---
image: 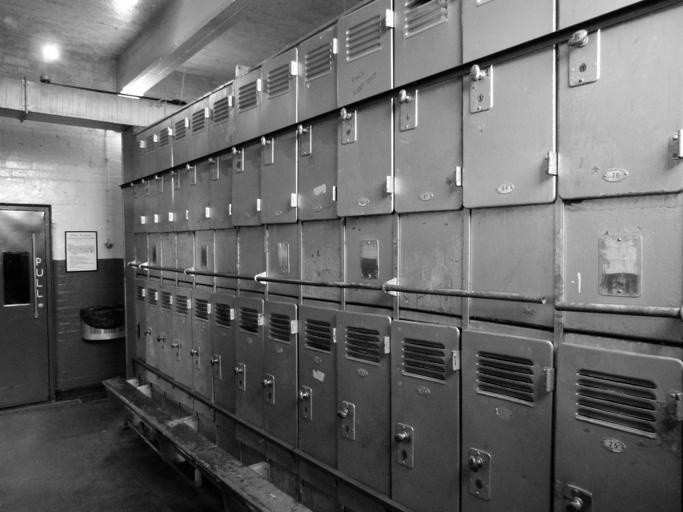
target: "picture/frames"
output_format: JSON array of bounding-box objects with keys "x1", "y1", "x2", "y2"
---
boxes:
[{"x1": 65, "y1": 229, "x2": 99, "y2": 273}]
